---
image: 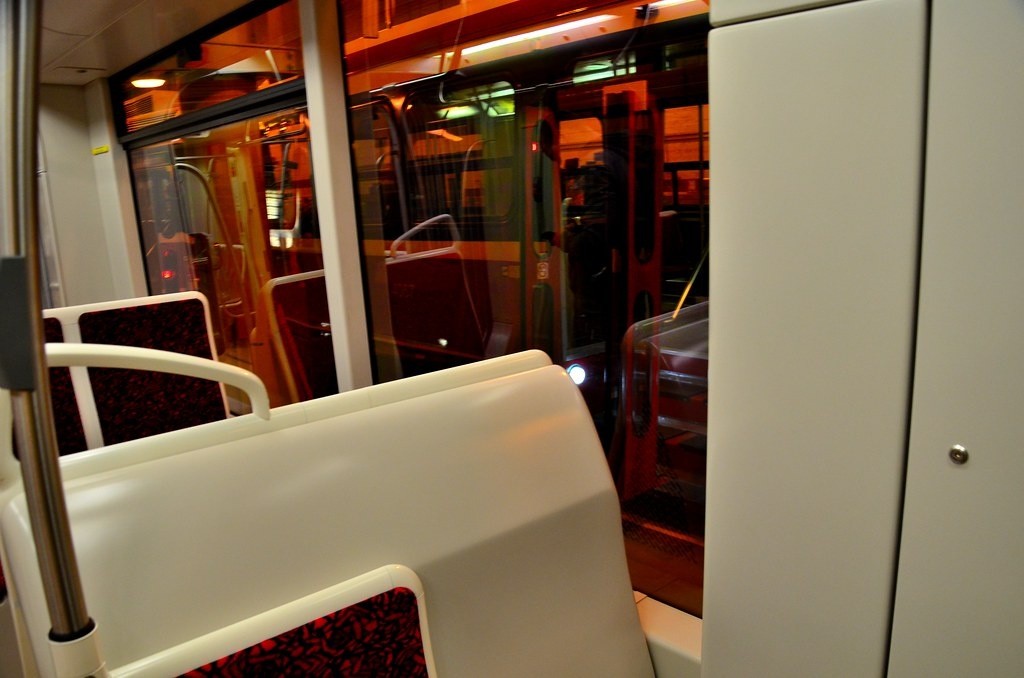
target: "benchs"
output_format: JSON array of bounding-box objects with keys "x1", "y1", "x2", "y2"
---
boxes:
[
  {"x1": 256, "y1": 214, "x2": 488, "y2": 412},
  {"x1": 11, "y1": 290, "x2": 231, "y2": 462},
  {"x1": 0, "y1": 346, "x2": 660, "y2": 678}
]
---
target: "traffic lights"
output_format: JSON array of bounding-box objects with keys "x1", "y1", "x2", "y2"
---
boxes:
[{"x1": 160, "y1": 233, "x2": 225, "y2": 355}]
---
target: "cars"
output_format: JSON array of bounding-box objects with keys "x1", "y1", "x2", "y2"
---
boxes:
[{"x1": 616, "y1": 248, "x2": 712, "y2": 451}]
